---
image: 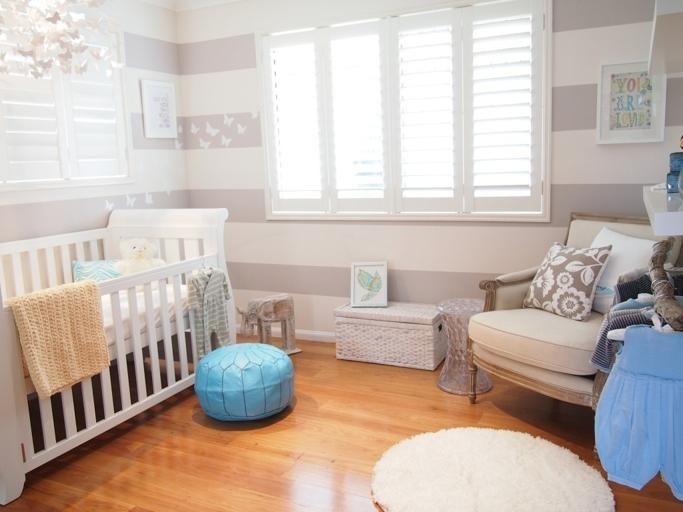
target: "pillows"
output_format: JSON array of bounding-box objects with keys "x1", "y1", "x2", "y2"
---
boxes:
[
  {"x1": 73, "y1": 259, "x2": 120, "y2": 281},
  {"x1": 522, "y1": 243, "x2": 612, "y2": 321},
  {"x1": 588, "y1": 225, "x2": 660, "y2": 315}
]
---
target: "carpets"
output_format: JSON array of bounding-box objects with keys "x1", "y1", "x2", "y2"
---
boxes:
[{"x1": 369, "y1": 428, "x2": 617, "y2": 512}]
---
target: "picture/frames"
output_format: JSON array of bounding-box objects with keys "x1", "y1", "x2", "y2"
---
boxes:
[
  {"x1": 595, "y1": 63, "x2": 667, "y2": 145},
  {"x1": 350, "y1": 262, "x2": 389, "y2": 307},
  {"x1": 142, "y1": 79, "x2": 178, "y2": 139}
]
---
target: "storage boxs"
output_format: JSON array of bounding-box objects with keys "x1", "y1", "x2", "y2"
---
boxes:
[{"x1": 334, "y1": 301, "x2": 447, "y2": 372}]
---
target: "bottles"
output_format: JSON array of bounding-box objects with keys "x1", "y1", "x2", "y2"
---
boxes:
[{"x1": 667, "y1": 152, "x2": 683, "y2": 210}]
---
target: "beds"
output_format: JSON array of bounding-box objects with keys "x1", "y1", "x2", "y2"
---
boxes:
[{"x1": 0, "y1": 208, "x2": 237, "y2": 505}]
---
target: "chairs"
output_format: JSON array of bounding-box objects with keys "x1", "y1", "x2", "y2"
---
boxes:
[{"x1": 467, "y1": 210, "x2": 683, "y2": 410}]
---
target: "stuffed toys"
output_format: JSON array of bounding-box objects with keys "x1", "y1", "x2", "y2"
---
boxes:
[{"x1": 113, "y1": 238, "x2": 158, "y2": 293}]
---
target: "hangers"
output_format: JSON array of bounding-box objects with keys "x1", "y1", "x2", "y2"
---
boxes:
[{"x1": 646, "y1": 238, "x2": 675, "y2": 326}]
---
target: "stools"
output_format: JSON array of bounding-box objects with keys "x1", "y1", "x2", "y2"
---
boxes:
[{"x1": 437, "y1": 299, "x2": 493, "y2": 394}]
